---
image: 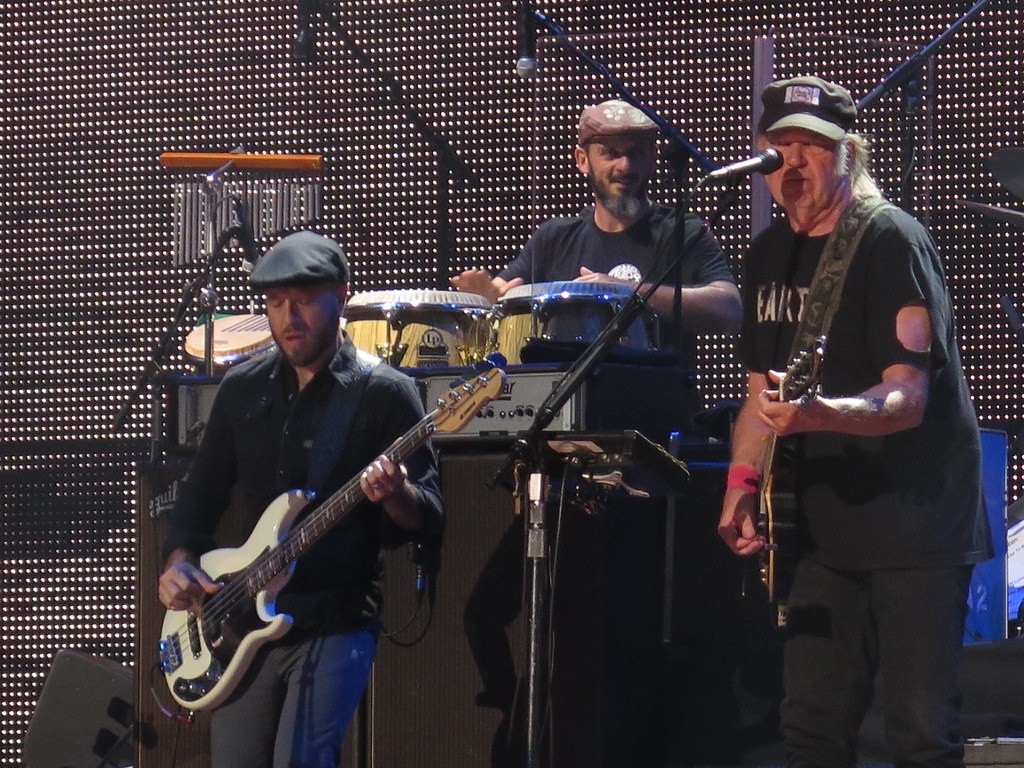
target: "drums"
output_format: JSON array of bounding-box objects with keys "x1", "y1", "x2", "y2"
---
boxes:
[
  {"x1": 491, "y1": 280, "x2": 659, "y2": 363},
  {"x1": 339, "y1": 288, "x2": 492, "y2": 370},
  {"x1": 182, "y1": 311, "x2": 275, "y2": 378}
]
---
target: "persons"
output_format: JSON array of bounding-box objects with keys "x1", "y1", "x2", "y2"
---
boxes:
[
  {"x1": 157, "y1": 231, "x2": 447, "y2": 768},
  {"x1": 448, "y1": 98, "x2": 744, "y2": 370},
  {"x1": 718, "y1": 75, "x2": 996, "y2": 767}
]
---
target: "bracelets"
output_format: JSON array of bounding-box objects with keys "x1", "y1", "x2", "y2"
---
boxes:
[{"x1": 727, "y1": 463, "x2": 762, "y2": 497}]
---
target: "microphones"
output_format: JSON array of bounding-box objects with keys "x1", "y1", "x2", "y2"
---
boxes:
[
  {"x1": 517, "y1": 0, "x2": 538, "y2": 79},
  {"x1": 707, "y1": 149, "x2": 784, "y2": 183},
  {"x1": 231, "y1": 196, "x2": 262, "y2": 272},
  {"x1": 294, "y1": 0, "x2": 317, "y2": 63}
]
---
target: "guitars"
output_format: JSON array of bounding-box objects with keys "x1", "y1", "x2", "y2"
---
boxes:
[
  {"x1": 733, "y1": 333, "x2": 829, "y2": 622},
  {"x1": 154, "y1": 353, "x2": 508, "y2": 713}
]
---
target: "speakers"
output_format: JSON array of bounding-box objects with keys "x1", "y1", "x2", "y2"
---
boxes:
[
  {"x1": 22, "y1": 649, "x2": 138, "y2": 768},
  {"x1": 136, "y1": 449, "x2": 606, "y2": 768}
]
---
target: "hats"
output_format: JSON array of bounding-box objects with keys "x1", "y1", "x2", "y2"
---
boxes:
[
  {"x1": 577, "y1": 98, "x2": 660, "y2": 145},
  {"x1": 247, "y1": 229, "x2": 351, "y2": 291},
  {"x1": 759, "y1": 76, "x2": 858, "y2": 141}
]
